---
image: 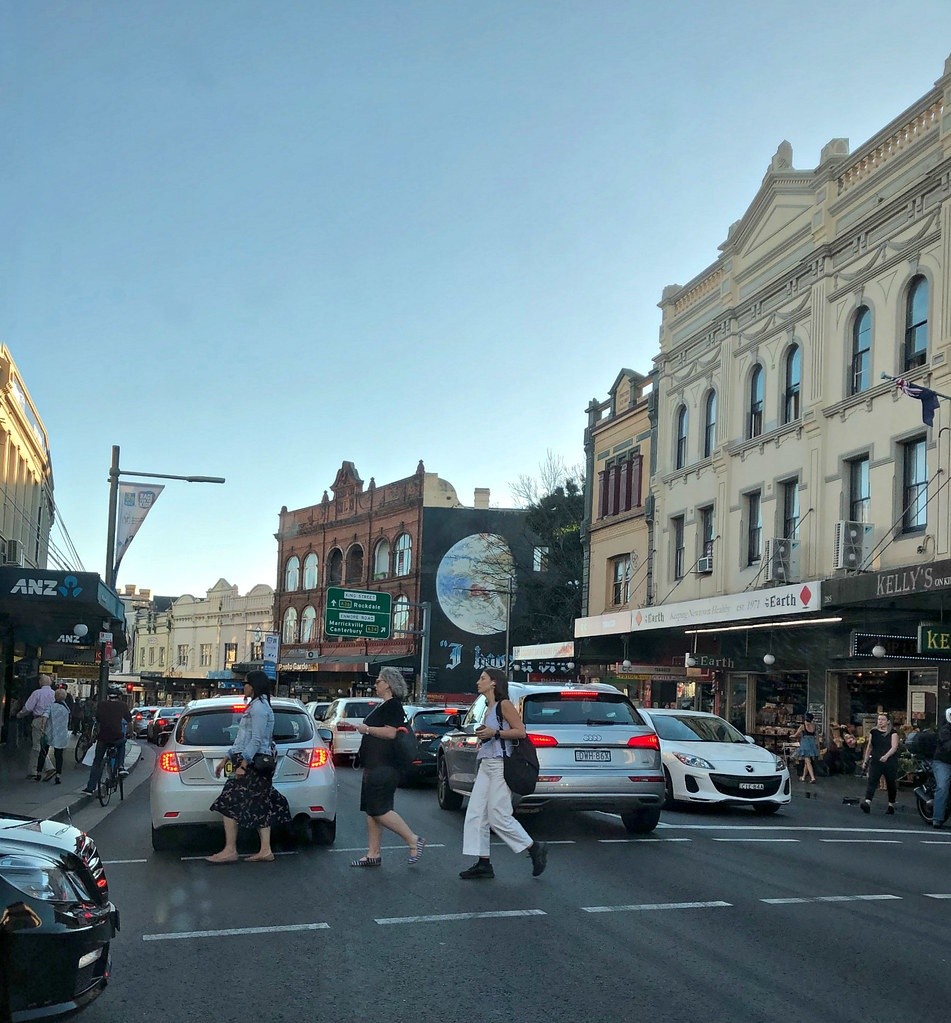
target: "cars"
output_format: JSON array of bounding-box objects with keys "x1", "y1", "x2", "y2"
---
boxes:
[
  {"x1": 0, "y1": 811, "x2": 121, "y2": 1023},
  {"x1": 315, "y1": 698, "x2": 385, "y2": 754},
  {"x1": 291, "y1": 701, "x2": 331, "y2": 735},
  {"x1": 402, "y1": 702, "x2": 472, "y2": 787},
  {"x1": 146, "y1": 707, "x2": 184, "y2": 746},
  {"x1": 130, "y1": 706, "x2": 160, "y2": 735},
  {"x1": 606, "y1": 708, "x2": 791, "y2": 813},
  {"x1": 149, "y1": 697, "x2": 338, "y2": 850}
]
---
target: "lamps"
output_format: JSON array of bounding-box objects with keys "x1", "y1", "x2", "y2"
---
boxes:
[
  {"x1": 687, "y1": 626, "x2": 696, "y2": 667},
  {"x1": 73, "y1": 616, "x2": 89, "y2": 637},
  {"x1": 623, "y1": 636, "x2": 631, "y2": 669},
  {"x1": 872, "y1": 604, "x2": 886, "y2": 658},
  {"x1": 763, "y1": 618, "x2": 775, "y2": 665}
]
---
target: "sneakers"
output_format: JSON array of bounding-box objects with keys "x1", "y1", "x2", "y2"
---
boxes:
[
  {"x1": 119, "y1": 767, "x2": 130, "y2": 775},
  {"x1": 459, "y1": 862, "x2": 495, "y2": 878},
  {"x1": 82, "y1": 788, "x2": 94, "y2": 795},
  {"x1": 526, "y1": 842, "x2": 548, "y2": 876}
]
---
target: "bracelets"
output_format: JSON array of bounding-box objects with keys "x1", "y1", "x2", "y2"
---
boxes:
[
  {"x1": 495, "y1": 730, "x2": 500, "y2": 740},
  {"x1": 240, "y1": 766, "x2": 247, "y2": 771},
  {"x1": 366, "y1": 728, "x2": 370, "y2": 735}
]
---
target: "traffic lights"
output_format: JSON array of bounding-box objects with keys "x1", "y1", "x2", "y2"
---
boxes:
[{"x1": 127, "y1": 684, "x2": 132, "y2": 694}]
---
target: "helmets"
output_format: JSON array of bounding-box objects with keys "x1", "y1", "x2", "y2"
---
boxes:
[{"x1": 945, "y1": 707, "x2": 951, "y2": 724}]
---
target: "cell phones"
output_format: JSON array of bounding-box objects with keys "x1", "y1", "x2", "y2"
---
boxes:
[{"x1": 475, "y1": 725, "x2": 491, "y2": 743}]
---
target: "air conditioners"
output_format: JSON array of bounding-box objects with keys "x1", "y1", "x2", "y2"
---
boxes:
[
  {"x1": 764, "y1": 538, "x2": 800, "y2": 585},
  {"x1": 697, "y1": 557, "x2": 713, "y2": 572},
  {"x1": 834, "y1": 520, "x2": 875, "y2": 572},
  {"x1": 305, "y1": 651, "x2": 318, "y2": 660},
  {"x1": 5, "y1": 540, "x2": 24, "y2": 568}
]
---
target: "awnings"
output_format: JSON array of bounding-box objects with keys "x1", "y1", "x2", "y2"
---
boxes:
[{"x1": 232, "y1": 654, "x2": 415, "y2": 673}]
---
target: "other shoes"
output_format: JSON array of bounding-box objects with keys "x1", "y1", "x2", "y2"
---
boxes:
[
  {"x1": 860, "y1": 802, "x2": 871, "y2": 813},
  {"x1": 798, "y1": 776, "x2": 805, "y2": 782},
  {"x1": 932, "y1": 818, "x2": 941, "y2": 829},
  {"x1": 809, "y1": 779, "x2": 816, "y2": 784},
  {"x1": 55, "y1": 776, "x2": 61, "y2": 784},
  {"x1": 408, "y1": 835, "x2": 426, "y2": 864},
  {"x1": 350, "y1": 855, "x2": 382, "y2": 865},
  {"x1": 886, "y1": 806, "x2": 896, "y2": 816},
  {"x1": 42, "y1": 770, "x2": 56, "y2": 781},
  {"x1": 243, "y1": 852, "x2": 274, "y2": 862},
  {"x1": 25, "y1": 774, "x2": 42, "y2": 782},
  {"x1": 205, "y1": 849, "x2": 239, "y2": 862}
]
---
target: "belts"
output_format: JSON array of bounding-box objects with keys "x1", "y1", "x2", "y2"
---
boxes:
[{"x1": 34, "y1": 715, "x2": 42, "y2": 718}]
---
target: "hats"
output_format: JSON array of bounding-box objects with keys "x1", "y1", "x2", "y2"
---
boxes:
[{"x1": 109, "y1": 689, "x2": 123, "y2": 698}]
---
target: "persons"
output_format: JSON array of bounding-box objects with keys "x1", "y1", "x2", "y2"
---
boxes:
[
  {"x1": 203, "y1": 670, "x2": 292, "y2": 863},
  {"x1": 81, "y1": 688, "x2": 132, "y2": 796},
  {"x1": 931, "y1": 707, "x2": 951, "y2": 828},
  {"x1": 458, "y1": 669, "x2": 547, "y2": 878},
  {"x1": 861, "y1": 713, "x2": 899, "y2": 815},
  {"x1": 17, "y1": 675, "x2": 83, "y2": 782},
  {"x1": 350, "y1": 666, "x2": 426, "y2": 866},
  {"x1": 790, "y1": 713, "x2": 820, "y2": 783}
]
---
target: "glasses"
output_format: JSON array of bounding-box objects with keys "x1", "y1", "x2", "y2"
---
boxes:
[
  {"x1": 375, "y1": 678, "x2": 387, "y2": 686},
  {"x1": 879, "y1": 711, "x2": 888, "y2": 716},
  {"x1": 241, "y1": 680, "x2": 250, "y2": 686}
]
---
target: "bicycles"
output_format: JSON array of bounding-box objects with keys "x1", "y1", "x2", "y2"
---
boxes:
[
  {"x1": 74, "y1": 715, "x2": 96, "y2": 762},
  {"x1": 97, "y1": 722, "x2": 123, "y2": 806}
]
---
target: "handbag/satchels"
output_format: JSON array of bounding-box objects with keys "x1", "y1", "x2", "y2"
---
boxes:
[
  {"x1": 389, "y1": 724, "x2": 420, "y2": 769},
  {"x1": 497, "y1": 697, "x2": 539, "y2": 796}
]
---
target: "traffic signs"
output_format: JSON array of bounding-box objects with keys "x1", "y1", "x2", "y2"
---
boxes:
[{"x1": 325, "y1": 587, "x2": 392, "y2": 640}]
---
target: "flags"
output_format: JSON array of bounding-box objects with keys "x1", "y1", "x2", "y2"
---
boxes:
[{"x1": 892, "y1": 376, "x2": 940, "y2": 426}]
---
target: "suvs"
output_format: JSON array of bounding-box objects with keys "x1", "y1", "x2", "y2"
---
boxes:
[{"x1": 437, "y1": 681, "x2": 665, "y2": 832}]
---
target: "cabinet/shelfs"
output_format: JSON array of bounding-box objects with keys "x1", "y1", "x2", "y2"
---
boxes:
[
  {"x1": 756, "y1": 674, "x2": 808, "y2": 705},
  {"x1": 845, "y1": 670, "x2": 887, "y2": 692}
]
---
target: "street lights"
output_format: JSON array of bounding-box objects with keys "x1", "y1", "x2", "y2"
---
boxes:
[
  {"x1": 98, "y1": 446, "x2": 225, "y2": 703},
  {"x1": 246, "y1": 630, "x2": 282, "y2": 698}
]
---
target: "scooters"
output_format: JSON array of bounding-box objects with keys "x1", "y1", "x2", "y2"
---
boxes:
[{"x1": 904, "y1": 733, "x2": 951, "y2": 824}]
